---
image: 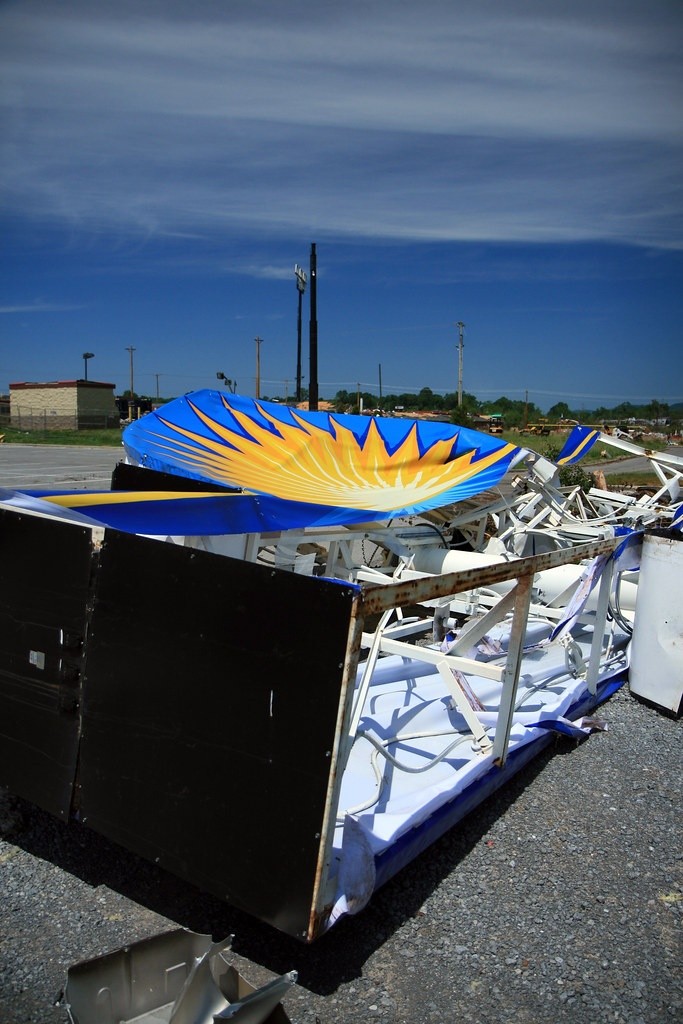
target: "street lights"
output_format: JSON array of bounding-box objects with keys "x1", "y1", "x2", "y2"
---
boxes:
[{"x1": 82, "y1": 352, "x2": 94, "y2": 382}]
